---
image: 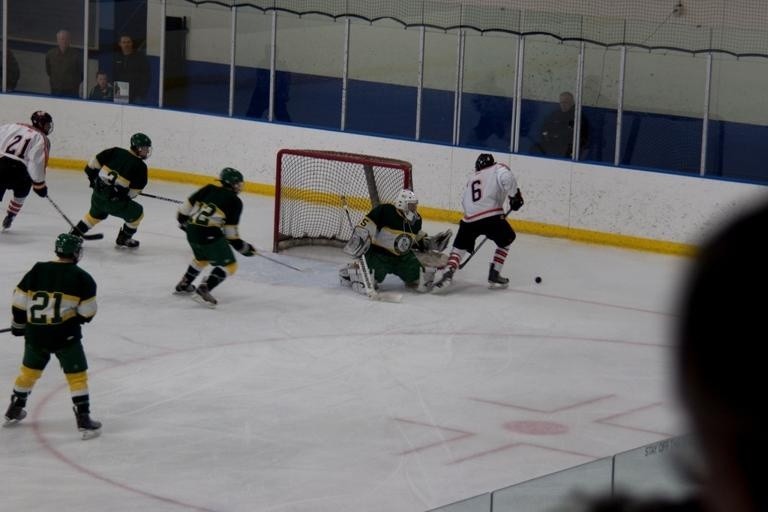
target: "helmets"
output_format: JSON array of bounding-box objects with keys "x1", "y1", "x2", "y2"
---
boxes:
[
  {"x1": 395, "y1": 189, "x2": 417, "y2": 221},
  {"x1": 131, "y1": 132, "x2": 151, "y2": 160},
  {"x1": 220, "y1": 167, "x2": 243, "y2": 189},
  {"x1": 55, "y1": 233, "x2": 83, "y2": 264},
  {"x1": 31, "y1": 110, "x2": 53, "y2": 134},
  {"x1": 475, "y1": 153, "x2": 494, "y2": 171}
]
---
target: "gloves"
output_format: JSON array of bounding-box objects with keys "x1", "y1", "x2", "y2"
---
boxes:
[
  {"x1": 239, "y1": 241, "x2": 254, "y2": 256},
  {"x1": 11, "y1": 321, "x2": 26, "y2": 336},
  {"x1": 33, "y1": 180, "x2": 47, "y2": 197},
  {"x1": 509, "y1": 188, "x2": 524, "y2": 211}
]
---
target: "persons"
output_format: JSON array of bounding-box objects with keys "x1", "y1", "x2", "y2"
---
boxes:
[
  {"x1": 174, "y1": 167, "x2": 255, "y2": 306},
  {"x1": 0, "y1": 51, "x2": 21, "y2": 91},
  {"x1": 112, "y1": 35, "x2": 151, "y2": 102},
  {"x1": 345, "y1": 186, "x2": 424, "y2": 285},
  {"x1": 438, "y1": 155, "x2": 526, "y2": 286},
  {"x1": 583, "y1": 192, "x2": 768, "y2": 512},
  {"x1": 532, "y1": 91, "x2": 586, "y2": 157},
  {"x1": 4, "y1": 233, "x2": 104, "y2": 433},
  {"x1": 45, "y1": 29, "x2": 86, "y2": 97},
  {"x1": 86, "y1": 73, "x2": 115, "y2": 101},
  {"x1": 0, "y1": 110, "x2": 53, "y2": 228},
  {"x1": 69, "y1": 131, "x2": 151, "y2": 248}
]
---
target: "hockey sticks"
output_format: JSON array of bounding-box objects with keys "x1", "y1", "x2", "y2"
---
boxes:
[
  {"x1": 46, "y1": 195, "x2": 103, "y2": 240},
  {"x1": 252, "y1": 251, "x2": 318, "y2": 275},
  {"x1": 436, "y1": 208, "x2": 512, "y2": 270},
  {"x1": 341, "y1": 196, "x2": 404, "y2": 302}
]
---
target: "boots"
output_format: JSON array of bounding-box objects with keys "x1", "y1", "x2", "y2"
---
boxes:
[
  {"x1": 487, "y1": 263, "x2": 508, "y2": 283},
  {"x1": 176, "y1": 276, "x2": 194, "y2": 292},
  {"x1": 5, "y1": 395, "x2": 26, "y2": 420},
  {"x1": 2, "y1": 213, "x2": 14, "y2": 228},
  {"x1": 69, "y1": 228, "x2": 80, "y2": 237},
  {"x1": 116, "y1": 227, "x2": 138, "y2": 247},
  {"x1": 436, "y1": 266, "x2": 455, "y2": 288},
  {"x1": 72, "y1": 406, "x2": 101, "y2": 429},
  {"x1": 196, "y1": 277, "x2": 216, "y2": 304}
]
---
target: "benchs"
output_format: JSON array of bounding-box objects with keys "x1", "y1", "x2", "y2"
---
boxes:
[{"x1": 98, "y1": 53, "x2": 722, "y2": 182}]
